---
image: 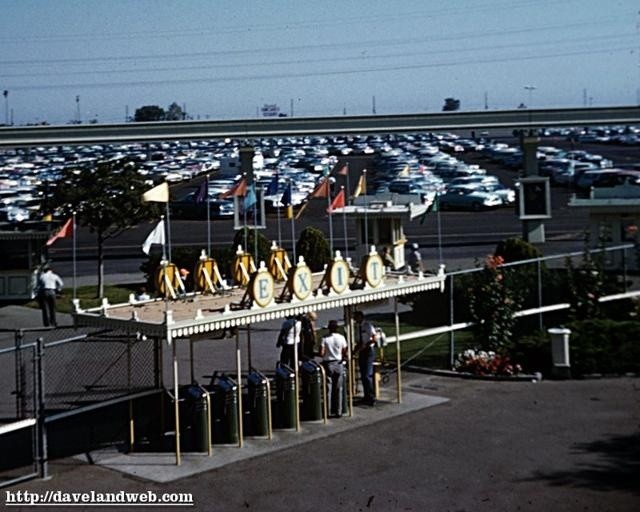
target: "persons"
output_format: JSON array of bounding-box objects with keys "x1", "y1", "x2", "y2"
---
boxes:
[
  {"x1": 351, "y1": 310, "x2": 376, "y2": 407},
  {"x1": 276, "y1": 312, "x2": 316, "y2": 369},
  {"x1": 26, "y1": 259, "x2": 64, "y2": 328},
  {"x1": 318, "y1": 320, "x2": 348, "y2": 418}
]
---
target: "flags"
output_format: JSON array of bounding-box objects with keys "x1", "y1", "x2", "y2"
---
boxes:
[
  {"x1": 44, "y1": 216, "x2": 73, "y2": 247},
  {"x1": 215, "y1": 175, "x2": 293, "y2": 219},
  {"x1": 141, "y1": 222, "x2": 165, "y2": 257},
  {"x1": 140, "y1": 181, "x2": 170, "y2": 205},
  {"x1": 311, "y1": 164, "x2": 365, "y2": 215},
  {"x1": 397, "y1": 163, "x2": 425, "y2": 177},
  {"x1": 191, "y1": 177, "x2": 208, "y2": 204}
]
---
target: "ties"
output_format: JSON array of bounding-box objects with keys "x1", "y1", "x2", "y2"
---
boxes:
[{"x1": 359, "y1": 324, "x2": 362, "y2": 342}]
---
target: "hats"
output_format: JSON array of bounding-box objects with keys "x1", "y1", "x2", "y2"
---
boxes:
[{"x1": 328, "y1": 320, "x2": 340, "y2": 329}]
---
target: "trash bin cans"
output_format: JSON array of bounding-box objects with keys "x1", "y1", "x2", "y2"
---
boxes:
[{"x1": 546, "y1": 327, "x2": 573, "y2": 367}]
